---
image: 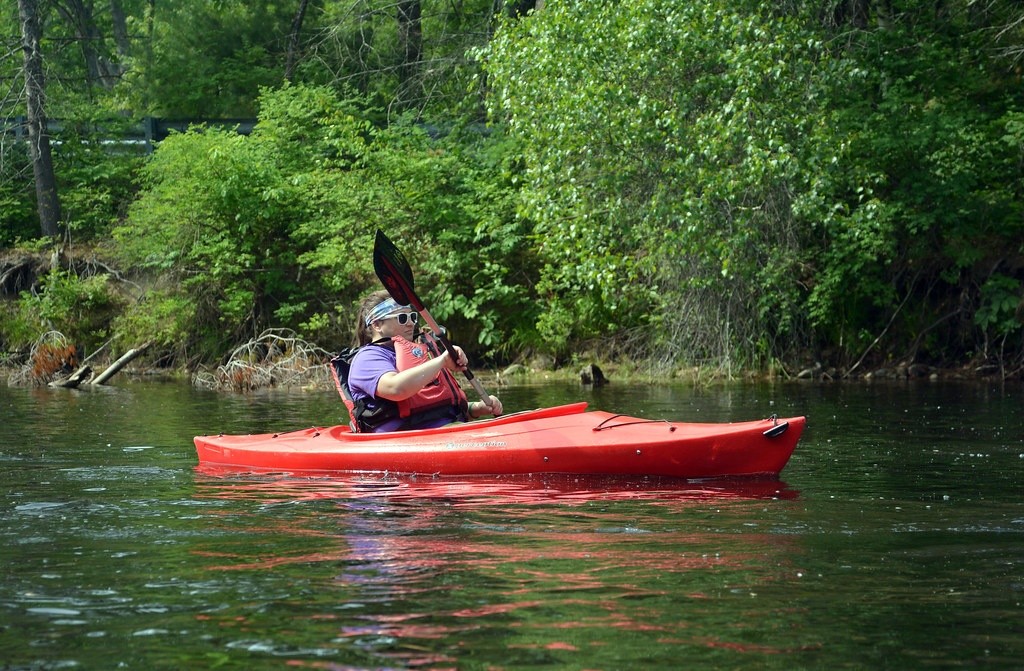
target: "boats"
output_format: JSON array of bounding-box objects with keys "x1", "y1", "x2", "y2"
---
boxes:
[{"x1": 193, "y1": 402, "x2": 806, "y2": 476}]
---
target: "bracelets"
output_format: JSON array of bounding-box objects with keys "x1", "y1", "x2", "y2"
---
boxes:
[{"x1": 468, "y1": 401, "x2": 480, "y2": 419}]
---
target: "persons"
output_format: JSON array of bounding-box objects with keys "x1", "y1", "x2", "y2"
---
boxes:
[{"x1": 345, "y1": 290, "x2": 501, "y2": 431}]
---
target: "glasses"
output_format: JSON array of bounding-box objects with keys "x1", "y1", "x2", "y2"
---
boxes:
[{"x1": 379, "y1": 312, "x2": 418, "y2": 326}]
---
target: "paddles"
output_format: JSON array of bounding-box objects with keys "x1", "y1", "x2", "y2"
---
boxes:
[{"x1": 372, "y1": 227, "x2": 498, "y2": 416}]
